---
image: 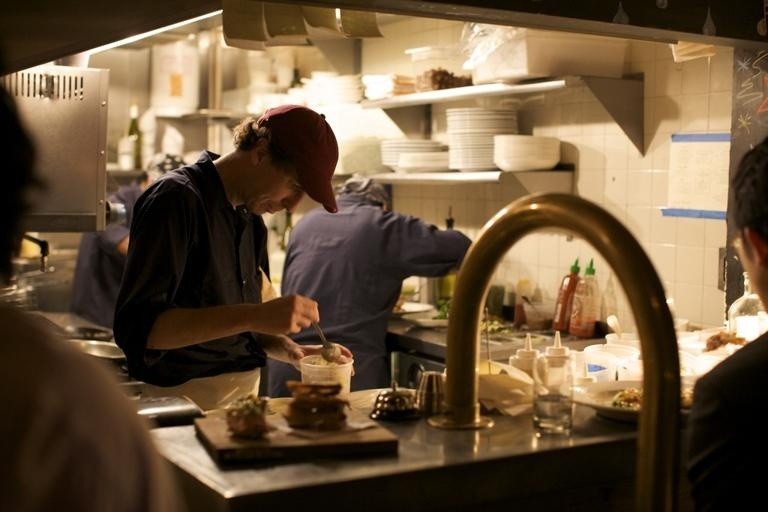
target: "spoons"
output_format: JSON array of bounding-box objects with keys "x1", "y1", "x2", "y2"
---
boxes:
[{"x1": 313, "y1": 322, "x2": 341, "y2": 362}]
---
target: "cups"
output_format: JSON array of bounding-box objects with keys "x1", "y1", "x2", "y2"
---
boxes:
[{"x1": 532, "y1": 356, "x2": 575, "y2": 438}]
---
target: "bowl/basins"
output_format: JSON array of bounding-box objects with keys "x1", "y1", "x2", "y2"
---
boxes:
[{"x1": 66, "y1": 327, "x2": 128, "y2": 368}]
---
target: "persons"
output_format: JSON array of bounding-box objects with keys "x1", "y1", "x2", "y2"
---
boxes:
[
  {"x1": 683, "y1": 136, "x2": 767, "y2": 510},
  {"x1": 111, "y1": 107, "x2": 358, "y2": 408},
  {"x1": 70, "y1": 152, "x2": 189, "y2": 326},
  {"x1": 263, "y1": 177, "x2": 474, "y2": 401},
  {"x1": 0, "y1": 82, "x2": 193, "y2": 512}
]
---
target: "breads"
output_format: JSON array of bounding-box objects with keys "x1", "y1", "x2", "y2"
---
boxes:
[
  {"x1": 225, "y1": 394, "x2": 268, "y2": 437},
  {"x1": 285, "y1": 395, "x2": 347, "y2": 429}
]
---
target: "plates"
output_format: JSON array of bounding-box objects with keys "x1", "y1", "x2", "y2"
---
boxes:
[
  {"x1": 571, "y1": 379, "x2": 695, "y2": 424},
  {"x1": 380, "y1": 106, "x2": 561, "y2": 173},
  {"x1": 400, "y1": 312, "x2": 448, "y2": 329}
]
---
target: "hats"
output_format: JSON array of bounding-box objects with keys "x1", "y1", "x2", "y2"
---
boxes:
[{"x1": 255, "y1": 104, "x2": 340, "y2": 214}]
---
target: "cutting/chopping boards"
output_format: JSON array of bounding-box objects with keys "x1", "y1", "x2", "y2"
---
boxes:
[{"x1": 194, "y1": 407, "x2": 399, "y2": 465}]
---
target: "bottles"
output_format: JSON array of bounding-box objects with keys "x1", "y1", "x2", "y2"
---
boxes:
[
  {"x1": 126, "y1": 104, "x2": 144, "y2": 168},
  {"x1": 551, "y1": 257, "x2": 599, "y2": 336},
  {"x1": 510, "y1": 330, "x2": 570, "y2": 385}
]
